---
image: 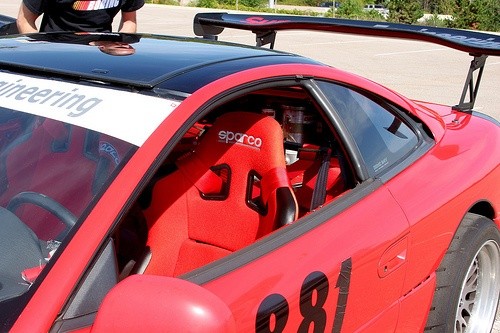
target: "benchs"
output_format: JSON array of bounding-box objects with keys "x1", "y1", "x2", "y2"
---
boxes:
[{"x1": 168, "y1": 93, "x2": 358, "y2": 219}]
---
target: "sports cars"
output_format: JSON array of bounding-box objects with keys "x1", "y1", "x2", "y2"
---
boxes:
[{"x1": 0, "y1": 9, "x2": 500, "y2": 333}]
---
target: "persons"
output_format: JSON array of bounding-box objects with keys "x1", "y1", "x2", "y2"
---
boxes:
[
  {"x1": 24, "y1": 31, "x2": 143, "y2": 56},
  {"x1": 16, "y1": 0, "x2": 145, "y2": 34}
]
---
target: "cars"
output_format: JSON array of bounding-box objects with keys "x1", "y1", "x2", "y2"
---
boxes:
[{"x1": 363, "y1": 4, "x2": 384, "y2": 15}]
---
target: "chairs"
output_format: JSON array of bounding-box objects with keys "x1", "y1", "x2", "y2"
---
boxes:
[
  {"x1": 142, "y1": 109, "x2": 298, "y2": 277},
  {"x1": 1, "y1": 118, "x2": 103, "y2": 245}
]
